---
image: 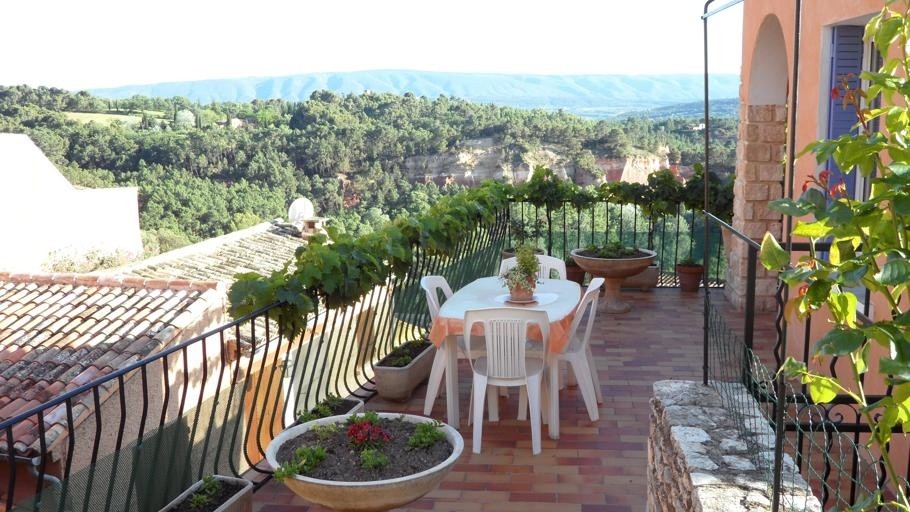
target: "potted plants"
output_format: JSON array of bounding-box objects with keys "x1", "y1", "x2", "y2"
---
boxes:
[
  {"x1": 265, "y1": 411, "x2": 465, "y2": 512},
  {"x1": 157, "y1": 470, "x2": 255, "y2": 512},
  {"x1": 501, "y1": 243, "x2": 542, "y2": 304},
  {"x1": 282, "y1": 395, "x2": 365, "y2": 434},
  {"x1": 572, "y1": 240, "x2": 657, "y2": 314},
  {"x1": 675, "y1": 255, "x2": 703, "y2": 293},
  {"x1": 564, "y1": 255, "x2": 586, "y2": 284},
  {"x1": 374, "y1": 338, "x2": 436, "y2": 403},
  {"x1": 501, "y1": 220, "x2": 546, "y2": 257}
]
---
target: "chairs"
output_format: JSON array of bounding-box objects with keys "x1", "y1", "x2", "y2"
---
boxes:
[
  {"x1": 497, "y1": 255, "x2": 569, "y2": 281},
  {"x1": 462, "y1": 307, "x2": 551, "y2": 457},
  {"x1": 518, "y1": 275, "x2": 607, "y2": 424},
  {"x1": 420, "y1": 274, "x2": 501, "y2": 429}
]
